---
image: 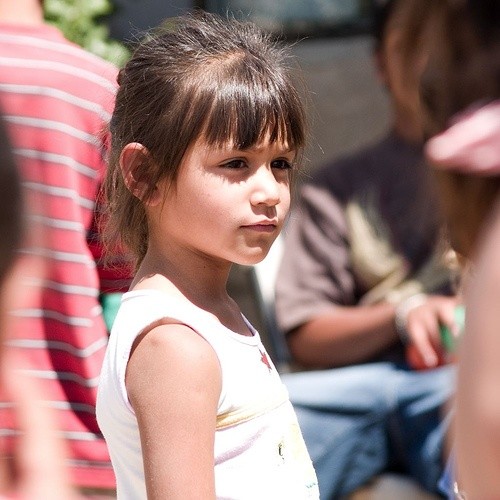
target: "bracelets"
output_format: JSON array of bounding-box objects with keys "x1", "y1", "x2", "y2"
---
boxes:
[{"x1": 395, "y1": 293, "x2": 425, "y2": 340}]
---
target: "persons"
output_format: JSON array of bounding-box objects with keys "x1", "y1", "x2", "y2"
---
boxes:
[
  {"x1": 91, "y1": 10, "x2": 325, "y2": 500},
  {"x1": 266, "y1": 0, "x2": 500, "y2": 500},
  {"x1": 1, "y1": 1, "x2": 129, "y2": 500}
]
